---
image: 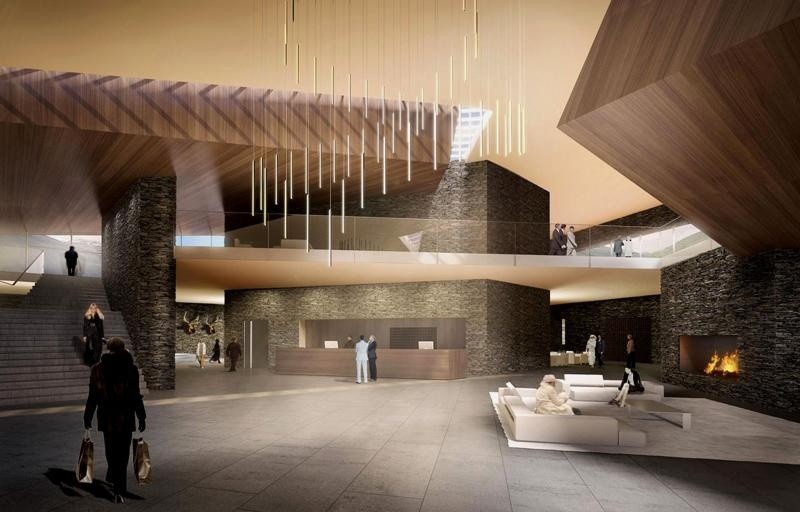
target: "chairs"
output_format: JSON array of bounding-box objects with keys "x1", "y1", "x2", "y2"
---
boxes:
[{"x1": 550, "y1": 351, "x2": 589, "y2": 367}]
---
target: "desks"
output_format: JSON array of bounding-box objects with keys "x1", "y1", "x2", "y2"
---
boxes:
[{"x1": 625, "y1": 400, "x2": 692, "y2": 432}]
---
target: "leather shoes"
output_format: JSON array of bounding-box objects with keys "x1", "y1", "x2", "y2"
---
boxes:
[{"x1": 114, "y1": 494, "x2": 127, "y2": 504}]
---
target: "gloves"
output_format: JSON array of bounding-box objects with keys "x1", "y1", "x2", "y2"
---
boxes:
[
  {"x1": 84, "y1": 419, "x2": 92, "y2": 430},
  {"x1": 138, "y1": 418, "x2": 146, "y2": 432}
]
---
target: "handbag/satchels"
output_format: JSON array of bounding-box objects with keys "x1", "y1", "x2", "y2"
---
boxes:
[
  {"x1": 73, "y1": 430, "x2": 95, "y2": 484},
  {"x1": 132, "y1": 430, "x2": 153, "y2": 488}
]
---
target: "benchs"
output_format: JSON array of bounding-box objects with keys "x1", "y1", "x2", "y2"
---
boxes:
[{"x1": 616, "y1": 417, "x2": 646, "y2": 447}]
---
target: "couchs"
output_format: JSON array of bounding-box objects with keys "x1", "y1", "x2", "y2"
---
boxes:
[
  {"x1": 497, "y1": 387, "x2": 618, "y2": 446},
  {"x1": 555, "y1": 373, "x2": 665, "y2": 401}
]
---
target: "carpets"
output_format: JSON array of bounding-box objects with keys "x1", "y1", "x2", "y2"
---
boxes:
[{"x1": 490, "y1": 391, "x2": 800, "y2": 465}]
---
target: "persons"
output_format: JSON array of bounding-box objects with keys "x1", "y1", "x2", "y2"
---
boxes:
[
  {"x1": 226, "y1": 336, "x2": 242, "y2": 372},
  {"x1": 65, "y1": 246, "x2": 78, "y2": 276},
  {"x1": 355, "y1": 335, "x2": 369, "y2": 384},
  {"x1": 624, "y1": 236, "x2": 633, "y2": 257},
  {"x1": 596, "y1": 334, "x2": 607, "y2": 368},
  {"x1": 196, "y1": 339, "x2": 207, "y2": 368},
  {"x1": 613, "y1": 235, "x2": 624, "y2": 257},
  {"x1": 83, "y1": 337, "x2": 146, "y2": 505},
  {"x1": 547, "y1": 223, "x2": 578, "y2": 256},
  {"x1": 367, "y1": 335, "x2": 378, "y2": 382},
  {"x1": 626, "y1": 334, "x2": 636, "y2": 368},
  {"x1": 84, "y1": 303, "x2": 107, "y2": 366},
  {"x1": 210, "y1": 339, "x2": 222, "y2": 364},
  {"x1": 534, "y1": 374, "x2": 576, "y2": 415},
  {"x1": 586, "y1": 334, "x2": 597, "y2": 368},
  {"x1": 344, "y1": 336, "x2": 354, "y2": 348},
  {"x1": 609, "y1": 366, "x2": 645, "y2": 408}
]
